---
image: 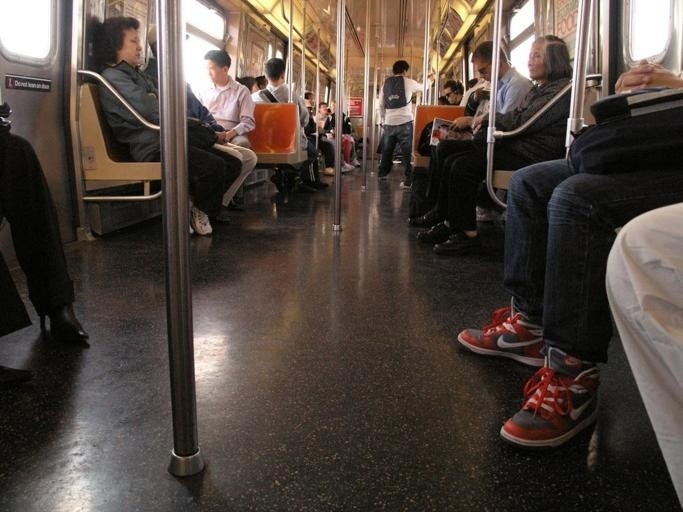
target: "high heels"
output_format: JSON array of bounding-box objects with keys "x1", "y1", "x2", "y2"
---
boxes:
[
  {"x1": 40, "y1": 305, "x2": 89, "y2": 342},
  {"x1": 1, "y1": 367, "x2": 36, "y2": 385}
]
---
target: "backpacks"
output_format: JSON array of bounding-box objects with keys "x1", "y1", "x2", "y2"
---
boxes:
[{"x1": 384, "y1": 76, "x2": 412, "y2": 110}]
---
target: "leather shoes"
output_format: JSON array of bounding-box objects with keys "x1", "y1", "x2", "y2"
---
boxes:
[{"x1": 408, "y1": 215, "x2": 482, "y2": 255}]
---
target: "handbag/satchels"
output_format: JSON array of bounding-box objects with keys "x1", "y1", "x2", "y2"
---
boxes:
[{"x1": 565, "y1": 86, "x2": 683, "y2": 176}]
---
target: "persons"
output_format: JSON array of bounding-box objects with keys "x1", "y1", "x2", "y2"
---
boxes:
[
  {"x1": 458, "y1": 24, "x2": 683, "y2": 451},
  {"x1": 376, "y1": 60, "x2": 435, "y2": 188},
  {"x1": 407, "y1": 34, "x2": 573, "y2": 255},
  {"x1": 1, "y1": 131, "x2": 90, "y2": 379},
  {"x1": 93, "y1": 16, "x2": 362, "y2": 234},
  {"x1": 606, "y1": 202, "x2": 683, "y2": 508}
]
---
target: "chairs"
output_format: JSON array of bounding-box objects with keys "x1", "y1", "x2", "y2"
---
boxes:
[
  {"x1": 352, "y1": 126, "x2": 370, "y2": 148},
  {"x1": 75, "y1": 81, "x2": 167, "y2": 201},
  {"x1": 248, "y1": 100, "x2": 309, "y2": 205},
  {"x1": 489, "y1": 86, "x2": 601, "y2": 192},
  {"x1": 410, "y1": 104, "x2": 465, "y2": 169}
]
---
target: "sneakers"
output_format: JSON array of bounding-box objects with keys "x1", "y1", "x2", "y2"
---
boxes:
[
  {"x1": 191, "y1": 206, "x2": 213, "y2": 236},
  {"x1": 299, "y1": 159, "x2": 360, "y2": 192},
  {"x1": 457, "y1": 297, "x2": 544, "y2": 368},
  {"x1": 499, "y1": 347, "x2": 600, "y2": 453}
]
